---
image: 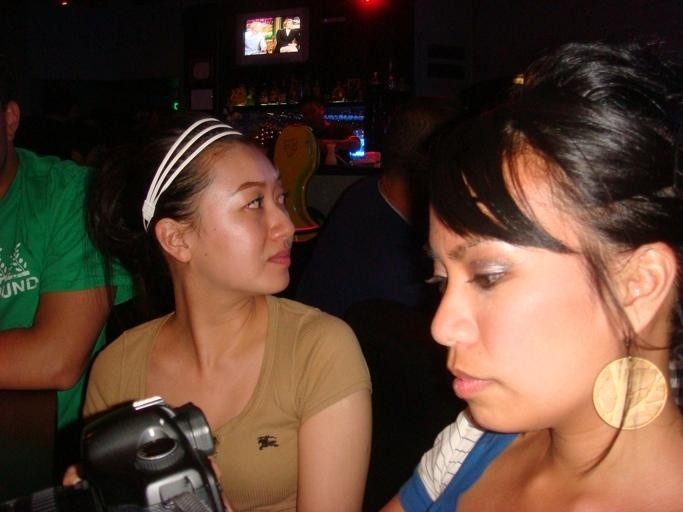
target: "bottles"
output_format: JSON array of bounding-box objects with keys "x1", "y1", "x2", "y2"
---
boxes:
[
  {"x1": 383, "y1": 63, "x2": 397, "y2": 90},
  {"x1": 254, "y1": 123, "x2": 275, "y2": 146},
  {"x1": 244, "y1": 77, "x2": 365, "y2": 105},
  {"x1": 324, "y1": 109, "x2": 366, "y2": 124},
  {"x1": 368, "y1": 71, "x2": 381, "y2": 86}
]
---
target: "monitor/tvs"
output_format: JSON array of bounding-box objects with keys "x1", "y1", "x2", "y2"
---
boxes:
[{"x1": 234, "y1": 6, "x2": 309, "y2": 67}]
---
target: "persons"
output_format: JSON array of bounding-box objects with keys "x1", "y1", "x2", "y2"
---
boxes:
[
  {"x1": 299, "y1": 94, "x2": 360, "y2": 166},
  {"x1": 60, "y1": 114, "x2": 374, "y2": 512},
  {"x1": 297, "y1": 72, "x2": 466, "y2": 512},
  {"x1": 0, "y1": 47, "x2": 135, "y2": 506},
  {"x1": 374, "y1": 29, "x2": 682, "y2": 512},
  {"x1": 94, "y1": 38, "x2": 188, "y2": 139}
]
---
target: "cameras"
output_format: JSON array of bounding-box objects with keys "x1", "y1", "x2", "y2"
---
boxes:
[{"x1": 0, "y1": 394, "x2": 227, "y2": 512}]
